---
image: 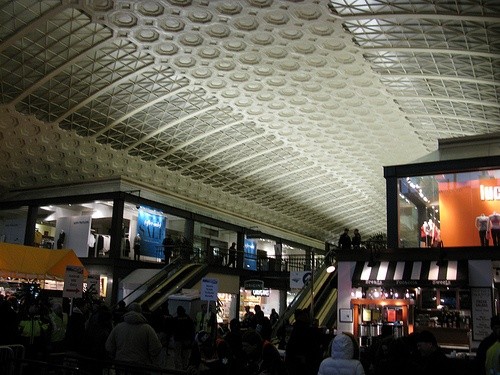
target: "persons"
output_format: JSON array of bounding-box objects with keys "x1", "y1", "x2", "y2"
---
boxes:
[
  {"x1": 289, "y1": 306, "x2": 329, "y2": 375},
  {"x1": 171, "y1": 306, "x2": 196, "y2": 373},
  {"x1": 196, "y1": 302, "x2": 278, "y2": 346},
  {"x1": 242, "y1": 330, "x2": 288, "y2": 375},
  {"x1": 18, "y1": 316, "x2": 49, "y2": 361},
  {"x1": 105, "y1": 303, "x2": 162, "y2": 375},
  {"x1": 124, "y1": 232, "x2": 130, "y2": 256},
  {"x1": 366, "y1": 316, "x2": 500, "y2": 375},
  {"x1": 339, "y1": 228, "x2": 351, "y2": 249},
  {"x1": 421, "y1": 219, "x2": 441, "y2": 247},
  {"x1": 133, "y1": 229, "x2": 145, "y2": 261},
  {"x1": 225, "y1": 242, "x2": 236, "y2": 268},
  {"x1": 475, "y1": 214, "x2": 490, "y2": 246},
  {"x1": 57, "y1": 229, "x2": 65, "y2": 249},
  {"x1": 159, "y1": 310, "x2": 173, "y2": 356},
  {"x1": 46, "y1": 303, "x2": 68, "y2": 356},
  {"x1": 318, "y1": 332, "x2": 365, "y2": 375},
  {"x1": 163, "y1": 233, "x2": 173, "y2": 264},
  {"x1": 352, "y1": 229, "x2": 361, "y2": 249},
  {"x1": 88, "y1": 230, "x2": 95, "y2": 257},
  {"x1": 44, "y1": 231, "x2": 48, "y2": 238},
  {"x1": 487, "y1": 211, "x2": 500, "y2": 246},
  {"x1": 64, "y1": 301, "x2": 89, "y2": 362},
  {"x1": 79, "y1": 314, "x2": 113, "y2": 375},
  {"x1": 0, "y1": 271, "x2": 127, "y2": 321}
]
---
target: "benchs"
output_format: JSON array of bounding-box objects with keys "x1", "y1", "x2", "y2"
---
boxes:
[{"x1": 415, "y1": 325, "x2": 473, "y2": 353}]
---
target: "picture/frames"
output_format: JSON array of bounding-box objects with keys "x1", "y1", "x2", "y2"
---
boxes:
[{"x1": 339, "y1": 308, "x2": 353, "y2": 323}]
